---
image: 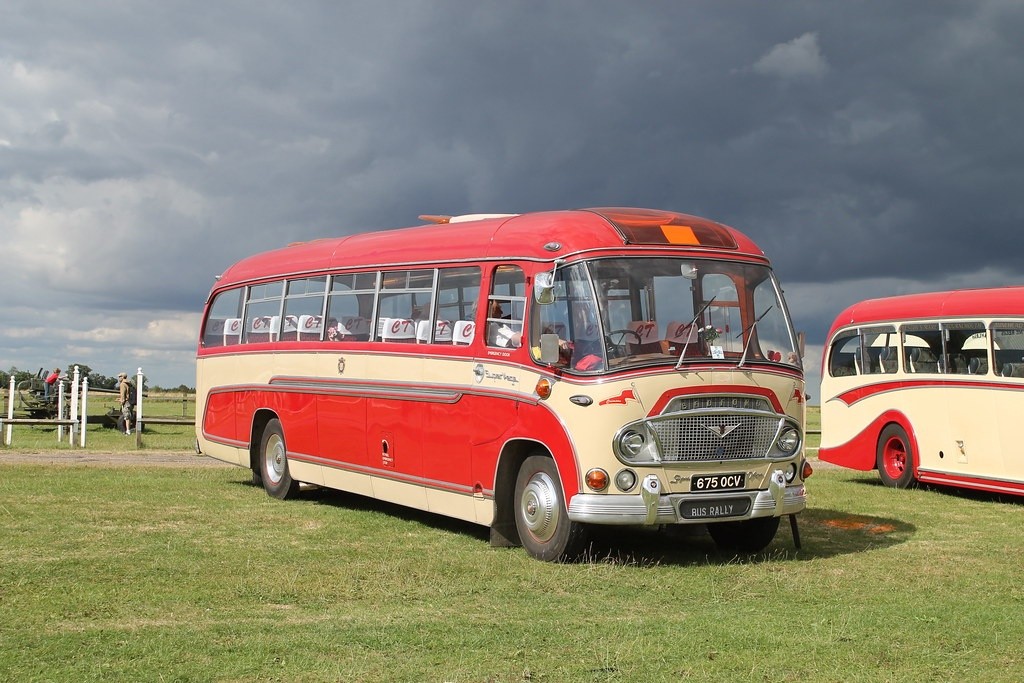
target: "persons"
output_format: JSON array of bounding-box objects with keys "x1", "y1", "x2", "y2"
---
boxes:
[
  {"x1": 42, "y1": 368, "x2": 61, "y2": 405},
  {"x1": 472, "y1": 298, "x2": 521, "y2": 348},
  {"x1": 116, "y1": 371, "x2": 138, "y2": 436}
]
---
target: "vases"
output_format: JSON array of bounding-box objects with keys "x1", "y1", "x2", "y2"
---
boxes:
[{"x1": 704, "y1": 339, "x2": 711, "y2": 356}]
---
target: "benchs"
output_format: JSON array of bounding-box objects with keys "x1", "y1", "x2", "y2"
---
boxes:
[
  {"x1": 1, "y1": 418, "x2": 80, "y2": 448},
  {"x1": 832, "y1": 356, "x2": 857, "y2": 377}
]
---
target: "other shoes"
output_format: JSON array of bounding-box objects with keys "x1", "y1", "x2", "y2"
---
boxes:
[{"x1": 123, "y1": 431, "x2": 131, "y2": 435}]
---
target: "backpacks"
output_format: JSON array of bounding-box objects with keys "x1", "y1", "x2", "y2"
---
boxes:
[{"x1": 124, "y1": 381, "x2": 137, "y2": 405}]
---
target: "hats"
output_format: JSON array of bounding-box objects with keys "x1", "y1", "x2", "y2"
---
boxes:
[{"x1": 117, "y1": 373, "x2": 127, "y2": 379}]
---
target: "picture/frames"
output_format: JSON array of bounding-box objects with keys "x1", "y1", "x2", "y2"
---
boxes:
[{"x1": 710, "y1": 346, "x2": 725, "y2": 359}]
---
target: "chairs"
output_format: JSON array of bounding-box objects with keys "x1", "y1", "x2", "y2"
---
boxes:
[
  {"x1": 327, "y1": 316, "x2": 344, "y2": 341},
  {"x1": 937, "y1": 353, "x2": 968, "y2": 374},
  {"x1": 574, "y1": 321, "x2": 603, "y2": 368},
  {"x1": 338, "y1": 316, "x2": 369, "y2": 342},
  {"x1": 378, "y1": 317, "x2": 391, "y2": 341},
  {"x1": 968, "y1": 357, "x2": 1001, "y2": 375},
  {"x1": 244, "y1": 315, "x2": 272, "y2": 343},
  {"x1": 878, "y1": 347, "x2": 910, "y2": 374},
  {"x1": 453, "y1": 320, "x2": 476, "y2": 346},
  {"x1": 1002, "y1": 362, "x2": 1024, "y2": 378},
  {"x1": 297, "y1": 315, "x2": 323, "y2": 342},
  {"x1": 854, "y1": 347, "x2": 881, "y2": 375},
  {"x1": 665, "y1": 321, "x2": 708, "y2": 355},
  {"x1": 416, "y1": 319, "x2": 452, "y2": 345},
  {"x1": 541, "y1": 320, "x2": 566, "y2": 340},
  {"x1": 204, "y1": 319, "x2": 229, "y2": 349},
  {"x1": 223, "y1": 318, "x2": 252, "y2": 346},
  {"x1": 624, "y1": 322, "x2": 664, "y2": 356},
  {"x1": 269, "y1": 315, "x2": 298, "y2": 342},
  {"x1": 909, "y1": 348, "x2": 939, "y2": 373},
  {"x1": 382, "y1": 318, "x2": 416, "y2": 344}
]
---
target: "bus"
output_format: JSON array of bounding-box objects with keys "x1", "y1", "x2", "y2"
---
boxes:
[
  {"x1": 817, "y1": 287, "x2": 1024, "y2": 498},
  {"x1": 193, "y1": 207, "x2": 815, "y2": 564}
]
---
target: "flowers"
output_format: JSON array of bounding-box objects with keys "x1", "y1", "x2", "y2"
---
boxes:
[{"x1": 699, "y1": 327, "x2": 723, "y2": 342}]
